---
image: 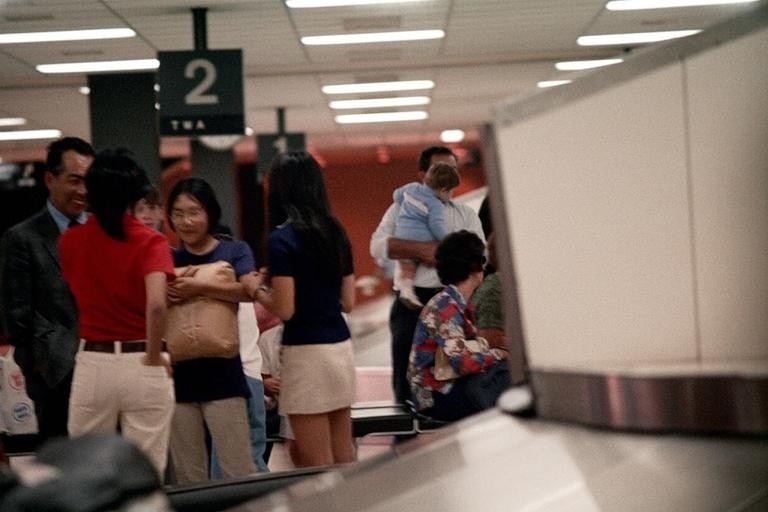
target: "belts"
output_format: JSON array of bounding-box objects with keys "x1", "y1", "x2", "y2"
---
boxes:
[{"x1": 83, "y1": 340, "x2": 168, "y2": 354}]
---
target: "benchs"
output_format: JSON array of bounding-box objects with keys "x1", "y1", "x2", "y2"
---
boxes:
[{"x1": 263, "y1": 399, "x2": 451, "y2": 467}]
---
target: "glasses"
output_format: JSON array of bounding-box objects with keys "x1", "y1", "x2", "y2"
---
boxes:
[{"x1": 170, "y1": 208, "x2": 206, "y2": 225}]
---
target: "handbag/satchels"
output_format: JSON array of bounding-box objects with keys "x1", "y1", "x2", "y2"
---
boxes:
[{"x1": 161, "y1": 260, "x2": 241, "y2": 365}]
